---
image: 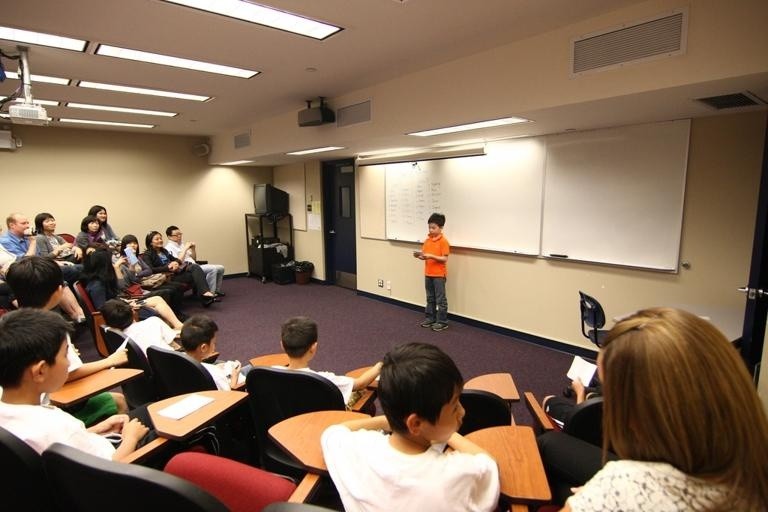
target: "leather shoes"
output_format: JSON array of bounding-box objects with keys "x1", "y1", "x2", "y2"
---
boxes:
[
  {"x1": 198, "y1": 291, "x2": 217, "y2": 298},
  {"x1": 199, "y1": 300, "x2": 212, "y2": 308}
]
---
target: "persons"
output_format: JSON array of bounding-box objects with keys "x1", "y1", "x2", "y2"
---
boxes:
[
  {"x1": 414, "y1": 213, "x2": 451, "y2": 332},
  {"x1": 542, "y1": 375, "x2": 603, "y2": 434},
  {"x1": 271, "y1": 314, "x2": 384, "y2": 413},
  {"x1": 1, "y1": 204, "x2": 233, "y2": 464},
  {"x1": 318, "y1": 339, "x2": 502, "y2": 512},
  {"x1": 558, "y1": 306, "x2": 768, "y2": 511}
]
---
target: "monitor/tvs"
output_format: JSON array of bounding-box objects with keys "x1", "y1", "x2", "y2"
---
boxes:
[{"x1": 252, "y1": 184, "x2": 288, "y2": 214}]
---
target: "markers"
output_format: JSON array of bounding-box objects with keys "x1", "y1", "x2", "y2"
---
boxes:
[{"x1": 549, "y1": 254, "x2": 568, "y2": 258}]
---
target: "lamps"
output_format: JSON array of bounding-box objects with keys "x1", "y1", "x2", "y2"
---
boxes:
[{"x1": 355, "y1": 139, "x2": 487, "y2": 167}]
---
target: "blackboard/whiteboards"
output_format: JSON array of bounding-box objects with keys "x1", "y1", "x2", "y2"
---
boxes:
[{"x1": 361, "y1": 118, "x2": 691, "y2": 275}]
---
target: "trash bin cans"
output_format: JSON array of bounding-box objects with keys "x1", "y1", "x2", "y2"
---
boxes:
[{"x1": 294, "y1": 270, "x2": 309, "y2": 284}]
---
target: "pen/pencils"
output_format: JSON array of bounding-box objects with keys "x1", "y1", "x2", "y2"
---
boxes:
[{"x1": 226, "y1": 364, "x2": 240, "y2": 379}]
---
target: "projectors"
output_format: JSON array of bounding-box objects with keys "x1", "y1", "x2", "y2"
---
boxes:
[{"x1": 9, "y1": 45, "x2": 52, "y2": 128}]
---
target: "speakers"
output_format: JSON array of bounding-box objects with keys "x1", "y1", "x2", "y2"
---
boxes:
[{"x1": 297, "y1": 107, "x2": 335, "y2": 126}]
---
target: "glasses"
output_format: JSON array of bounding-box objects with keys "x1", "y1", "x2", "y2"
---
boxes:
[{"x1": 171, "y1": 234, "x2": 182, "y2": 236}]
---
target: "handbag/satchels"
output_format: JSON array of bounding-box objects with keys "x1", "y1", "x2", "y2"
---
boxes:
[
  {"x1": 141, "y1": 274, "x2": 165, "y2": 289},
  {"x1": 125, "y1": 285, "x2": 143, "y2": 295},
  {"x1": 56, "y1": 248, "x2": 82, "y2": 264}
]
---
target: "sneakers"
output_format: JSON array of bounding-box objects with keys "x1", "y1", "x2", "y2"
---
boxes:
[
  {"x1": 433, "y1": 322, "x2": 449, "y2": 331},
  {"x1": 214, "y1": 298, "x2": 222, "y2": 302},
  {"x1": 215, "y1": 290, "x2": 225, "y2": 296},
  {"x1": 420, "y1": 319, "x2": 436, "y2": 327}
]
